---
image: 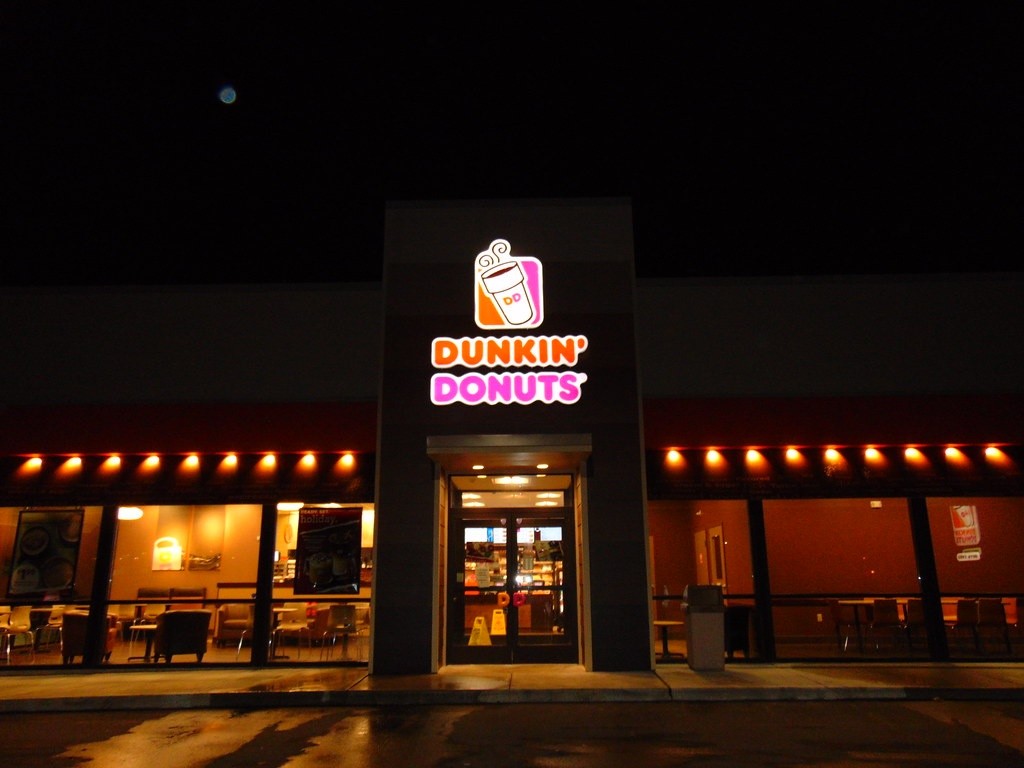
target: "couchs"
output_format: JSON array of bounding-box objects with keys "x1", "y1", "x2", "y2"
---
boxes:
[
  {"x1": 62, "y1": 610, "x2": 117, "y2": 664},
  {"x1": 152, "y1": 609, "x2": 212, "y2": 663},
  {"x1": 215, "y1": 604, "x2": 255, "y2": 649}
]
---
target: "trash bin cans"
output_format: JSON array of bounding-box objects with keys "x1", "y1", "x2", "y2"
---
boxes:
[{"x1": 681, "y1": 582, "x2": 727, "y2": 670}]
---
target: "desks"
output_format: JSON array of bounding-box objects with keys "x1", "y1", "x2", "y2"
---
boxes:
[
  {"x1": 654, "y1": 620, "x2": 685, "y2": 661},
  {"x1": 270, "y1": 608, "x2": 298, "y2": 660},
  {"x1": 128, "y1": 625, "x2": 165, "y2": 662},
  {"x1": 840, "y1": 600, "x2": 1010, "y2": 646}
]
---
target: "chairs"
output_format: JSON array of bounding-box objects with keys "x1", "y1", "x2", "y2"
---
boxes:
[
  {"x1": 724, "y1": 604, "x2": 752, "y2": 659},
  {"x1": 830, "y1": 598, "x2": 1011, "y2": 652},
  {"x1": 236, "y1": 601, "x2": 368, "y2": 661},
  {"x1": 0, "y1": 606, "x2": 89, "y2": 664},
  {"x1": 110, "y1": 615, "x2": 135, "y2": 642}
]
